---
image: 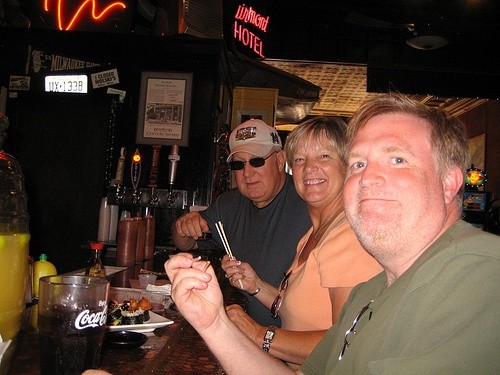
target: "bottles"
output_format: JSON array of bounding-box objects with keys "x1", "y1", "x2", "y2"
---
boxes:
[
  {"x1": 30, "y1": 254, "x2": 57, "y2": 331},
  {"x1": 116, "y1": 211, "x2": 155, "y2": 266},
  {"x1": 84, "y1": 242, "x2": 106, "y2": 279},
  {"x1": 0, "y1": 113, "x2": 31, "y2": 375}
]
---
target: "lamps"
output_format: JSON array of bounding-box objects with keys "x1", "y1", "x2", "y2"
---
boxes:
[{"x1": 406, "y1": 0, "x2": 459, "y2": 50}]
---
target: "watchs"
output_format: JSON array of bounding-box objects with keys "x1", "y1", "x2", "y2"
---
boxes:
[{"x1": 262, "y1": 325, "x2": 277, "y2": 354}]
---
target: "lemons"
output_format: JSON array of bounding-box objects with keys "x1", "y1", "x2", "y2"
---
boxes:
[{"x1": 32, "y1": 254, "x2": 57, "y2": 296}]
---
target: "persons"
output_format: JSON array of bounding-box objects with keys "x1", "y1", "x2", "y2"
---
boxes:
[
  {"x1": 173, "y1": 117, "x2": 311, "y2": 327},
  {"x1": 164, "y1": 92, "x2": 500, "y2": 375},
  {"x1": 221, "y1": 114, "x2": 384, "y2": 369}
]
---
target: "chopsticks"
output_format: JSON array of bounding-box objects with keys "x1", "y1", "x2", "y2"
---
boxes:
[
  {"x1": 215, "y1": 221, "x2": 244, "y2": 290},
  {"x1": 193, "y1": 255, "x2": 210, "y2": 272}
]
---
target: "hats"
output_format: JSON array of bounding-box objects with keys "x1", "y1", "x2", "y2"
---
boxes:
[{"x1": 225, "y1": 118, "x2": 282, "y2": 163}]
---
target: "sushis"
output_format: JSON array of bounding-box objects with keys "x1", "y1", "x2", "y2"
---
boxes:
[{"x1": 107, "y1": 297, "x2": 153, "y2": 325}]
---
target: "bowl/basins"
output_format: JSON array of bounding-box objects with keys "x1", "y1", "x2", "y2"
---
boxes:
[{"x1": 104, "y1": 331, "x2": 148, "y2": 351}]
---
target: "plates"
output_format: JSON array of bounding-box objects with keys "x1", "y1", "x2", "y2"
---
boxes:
[{"x1": 106, "y1": 303, "x2": 175, "y2": 333}]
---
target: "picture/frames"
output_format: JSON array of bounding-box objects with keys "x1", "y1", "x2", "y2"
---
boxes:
[{"x1": 136, "y1": 70, "x2": 194, "y2": 146}]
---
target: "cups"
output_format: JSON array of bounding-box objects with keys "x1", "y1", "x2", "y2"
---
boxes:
[
  {"x1": 138, "y1": 274, "x2": 157, "y2": 288},
  {"x1": 98, "y1": 197, "x2": 129, "y2": 243},
  {"x1": 38, "y1": 275, "x2": 110, "y2": 375}
]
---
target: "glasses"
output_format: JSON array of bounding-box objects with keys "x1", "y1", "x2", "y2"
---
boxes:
[
  {"x1": 230, "y1": 150, "x2": 278, "y2": 170},
  {"x1": 337, "y1": 299, "x2": 374, "y2": 360},
  {"x1": 270, "y1": 269, "x2": 293, "y2": 319}
]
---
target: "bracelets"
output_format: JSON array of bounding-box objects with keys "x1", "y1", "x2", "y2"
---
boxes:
[{"x1": 250, "y1": 287, "x2": 261, "y2": 297}]
